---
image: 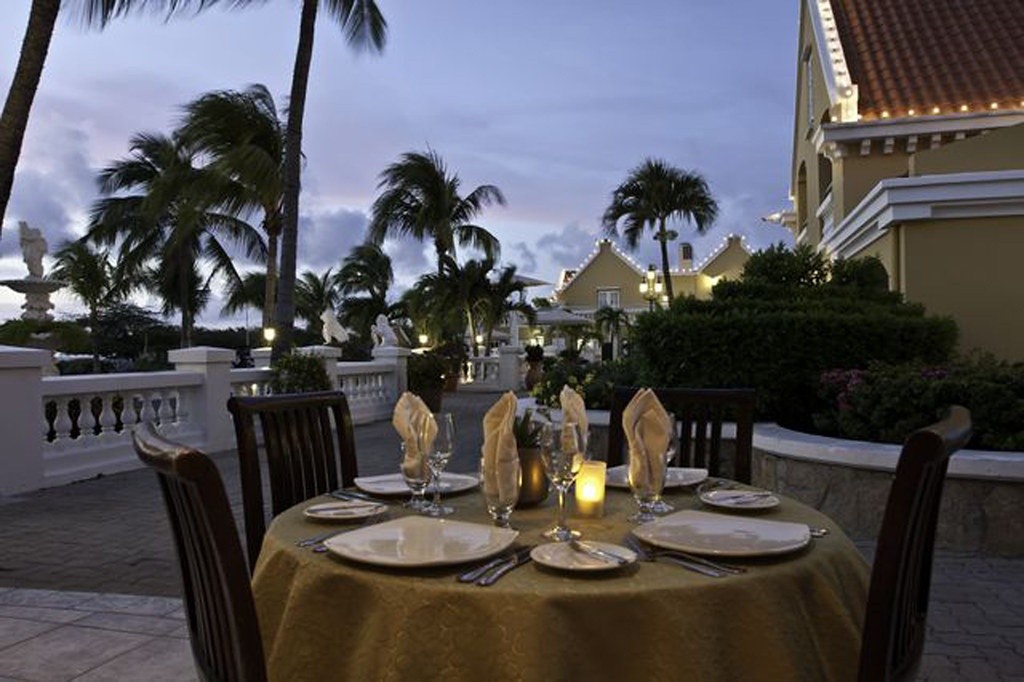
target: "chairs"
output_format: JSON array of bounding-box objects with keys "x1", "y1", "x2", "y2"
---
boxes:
[
  {"x1": 603, "y1": 384, "x2": 756, "y2": 490},
  {"x1": 225, "y1": 389, "x2": 361, "y2": 579},
  {"x1": 859, "y1": 406, "x2": 973, "y2": 682},
  {"x1": 131, "y1": 421, "x2": 269, "y2": 682}
]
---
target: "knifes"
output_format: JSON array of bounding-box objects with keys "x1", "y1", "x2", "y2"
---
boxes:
[{"x1": 458, "y1": 544, "x2": 538, "y2": 585}]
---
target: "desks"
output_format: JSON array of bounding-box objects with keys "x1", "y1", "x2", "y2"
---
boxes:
[{"x1": 252, "y1": 473, "x2": 872, "y2": 682}]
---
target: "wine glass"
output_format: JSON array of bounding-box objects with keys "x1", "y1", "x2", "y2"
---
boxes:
[
  {"x1": 478, "y1": 458, "x2": 519, "y2": 530},
  {"x1": 399, "y1": 442, "x2": 431, "y2": 510},
  {"x1": 540, "y1": 423, "x2": 587, "y2": 541},
  {"x1": 422, "y1": 412, "x2": 455, "y2": 516},
  {"x1": 628, "y1": 453, "x2": 667, "y2": 523}
]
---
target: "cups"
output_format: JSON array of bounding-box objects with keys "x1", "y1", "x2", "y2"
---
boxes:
[{"x1": 666, "y1": 412, "x2": 677, "y2": 462}]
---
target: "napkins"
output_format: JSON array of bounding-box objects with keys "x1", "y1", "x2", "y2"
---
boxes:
[{"x1": 391, "y1": 380, "x2": 672, "y2": 509}]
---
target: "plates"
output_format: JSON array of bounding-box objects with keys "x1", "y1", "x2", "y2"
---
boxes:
[
  {"x1": 304, "y1": 501, "x2": 388, "y2": 519},
  {"x1": 323, "y1": 515, "x2": 520, "y2": 567},
  {"x1": 630, "y1": 509, "x2": 810, "y2": 556},
  {"x1": 529, "y1": 540, "x2": 637, "y2": 569},
  {"x1": 605, "y1": 464, "x2": 708, "y2": 487},
  {"x1": 354, "y1": 471, "x2": 480, "y2": 494},
  {"x1": 701, "y1": 491, "x2": 780, "y2": 508}
]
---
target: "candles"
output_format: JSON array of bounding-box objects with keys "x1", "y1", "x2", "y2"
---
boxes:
[{"x1": 576, "y1": 460, "x2": 607, "y2": 519}]
